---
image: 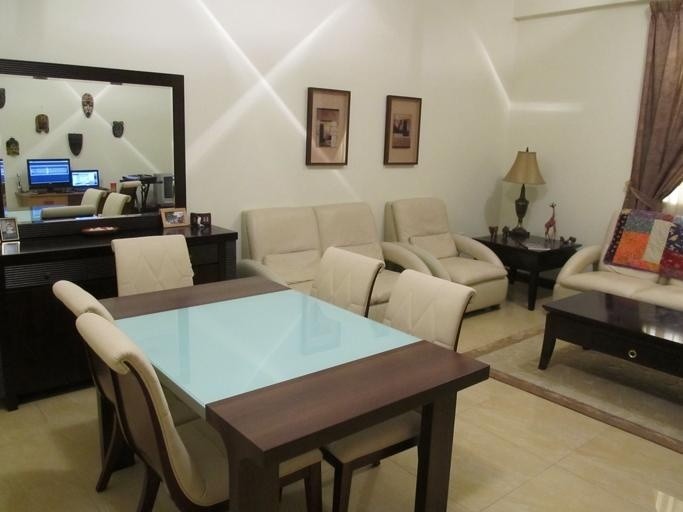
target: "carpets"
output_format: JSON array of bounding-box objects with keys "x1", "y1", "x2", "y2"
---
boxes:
[{"x1": 456, "y1": 273, "x2": 683, "y2": 456}]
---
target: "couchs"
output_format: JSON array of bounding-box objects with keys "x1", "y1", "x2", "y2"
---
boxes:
[
  {"x1": 237, "y1": 200, "x2": 432, "y2": 322},
  {"x1": 552, "y1": 242, "x2": 683, "y2": 312}
]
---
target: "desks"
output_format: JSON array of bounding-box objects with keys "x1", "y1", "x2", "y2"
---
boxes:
[
  {"x1": 475, "y1": 229, "x2": 582, "y2": 312},
  {"x1": 15, "y1": 186, "x2": 111, "y2": 206},
  {"x1": 120, "y1": 178, "x2": 163, "y2": 213}
]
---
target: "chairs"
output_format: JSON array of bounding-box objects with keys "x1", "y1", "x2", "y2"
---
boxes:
[
  {"x1": 119, "y1": 180, "x2": 142, "y2": 214},
  {"x1": 81, "y1": 188, "x2": 107, "y2": 208},
  {"x1": 383, "y1": 198, "x2": 509, "y2": 318},
  {"x1": 41, "y1": 205, "x2": 97, "y2": 218},
  {"x1": 102, "y1": 191, "x2": 132, "y2": 217}
]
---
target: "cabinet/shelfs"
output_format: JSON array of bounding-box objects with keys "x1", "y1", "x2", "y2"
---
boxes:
[{"x1": 0, "y1": 222, "x2": 238, "y2": 412}]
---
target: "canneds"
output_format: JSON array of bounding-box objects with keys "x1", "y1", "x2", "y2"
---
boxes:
[{"x1": 111, "y1": 182, "x2": 116, "y2": 192}]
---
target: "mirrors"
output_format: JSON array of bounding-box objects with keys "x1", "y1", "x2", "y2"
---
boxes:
[{"x1": 0, "y1": 58, "x2": 188, "y2": 238}]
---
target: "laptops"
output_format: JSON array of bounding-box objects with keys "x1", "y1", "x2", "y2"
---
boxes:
[{"x1": 73, "y1": 170, "x2": 108, "y2": 192}]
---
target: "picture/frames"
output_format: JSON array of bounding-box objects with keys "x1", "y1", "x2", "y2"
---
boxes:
[
  {"x1": 305, "y1": 87, "x2": 351, "y2": 166},
  {"x1": 384, "y1": 95, "x2": 422, "y2": 165},
  {"x1": 0, "y1": 218, "x2": 20, "y2": 241},
  {"x1": 159, "y1": 207, "x2": 211, "y2": 231},
  {"x1": 1, "y1": 241, "x2": 20, "y2": 256}
]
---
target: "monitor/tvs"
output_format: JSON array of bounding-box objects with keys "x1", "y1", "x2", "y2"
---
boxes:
[{"x1": 26, "y1": 159, "x2": 71, "y2": 192}]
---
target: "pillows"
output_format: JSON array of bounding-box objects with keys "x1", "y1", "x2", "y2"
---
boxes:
[
  {"x1": 597, "y1": 207, "x2": 675, "y2": 284},
  {"x1": 658, "y1": 214, "x2": 682, "y2": 289}
]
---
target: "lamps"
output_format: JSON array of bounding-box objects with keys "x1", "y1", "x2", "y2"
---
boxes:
[{"x1": 502, "y1": 147, "x2": 545, "y2": 238}]
